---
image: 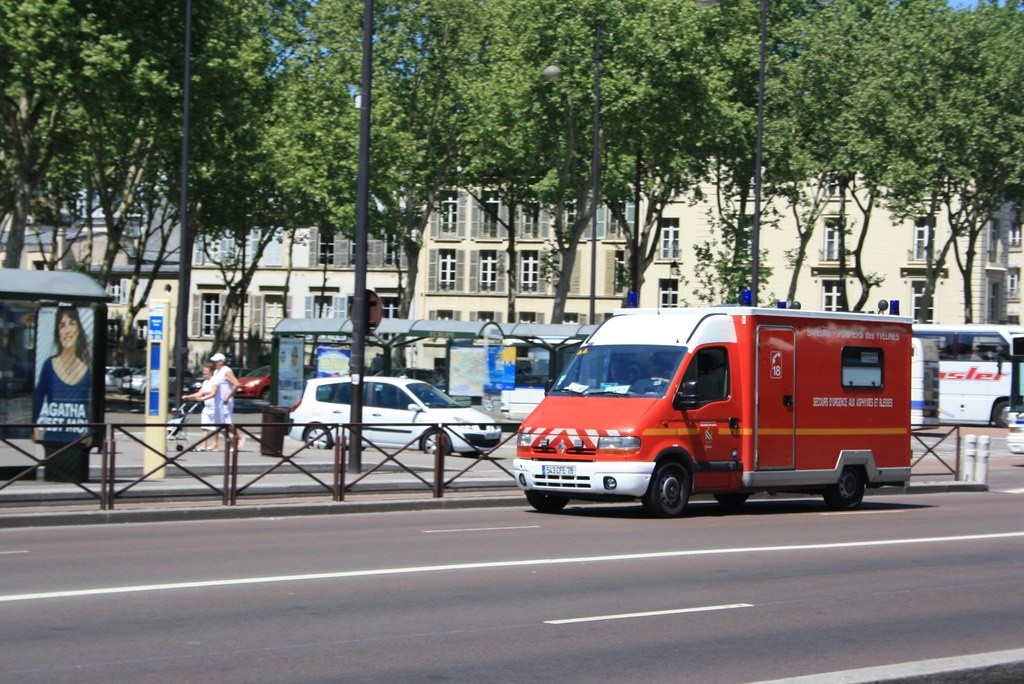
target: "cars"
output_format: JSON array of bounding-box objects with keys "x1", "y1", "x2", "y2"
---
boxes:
[
  {"x1": 188, "y1": 367, "x2": 251, "y2": 393},
  {"x1": 233, "y1": 364, "x2": 270, "y2": 400},
  {"x1": 118, "y1": 365, "x2": 195, "y2": 396}
]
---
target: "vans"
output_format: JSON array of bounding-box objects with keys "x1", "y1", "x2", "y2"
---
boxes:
[{"x1": 512, "y1": 288, "x2": 917, "y2": 518}]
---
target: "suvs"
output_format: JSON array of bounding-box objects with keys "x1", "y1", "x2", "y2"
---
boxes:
[
  {"x1": 290, "y1": 375, "x2": 498, "y2": 462},
  {"x1": 105, "y1": 366, "x2": 140, "y2": 395}
]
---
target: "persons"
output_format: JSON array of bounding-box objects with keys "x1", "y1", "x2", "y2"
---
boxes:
[
  {"x1": 33, "y1": 306, "x2": 93, "y2": 443},
  {"x1": 182, "y1": 352, "x2": 246, "y2": 453}
]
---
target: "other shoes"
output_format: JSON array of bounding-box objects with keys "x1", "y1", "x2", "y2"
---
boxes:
[
  {"x1": 230, "y1": 436, "x2": 245, "y2": 453},
  {"x1": 193, "y1": 448, "x2": 206, "y2": 452},
  {"x1": 207, "y1": 448, "x2": 219, "y2": 452}
]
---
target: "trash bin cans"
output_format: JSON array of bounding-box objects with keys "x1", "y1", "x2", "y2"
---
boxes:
[{"x1": 257, "y1": 401, "x2": 295, "y2": 457}]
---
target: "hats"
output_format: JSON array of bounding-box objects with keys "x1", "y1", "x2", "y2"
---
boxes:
[{"x1": 210, "y1": 353, "x2": 226, "y2": 362}]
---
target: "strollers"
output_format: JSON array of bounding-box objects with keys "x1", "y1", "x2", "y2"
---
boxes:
[{"x1": 166, "y1": 398, "x2": 201, "y2": 452}]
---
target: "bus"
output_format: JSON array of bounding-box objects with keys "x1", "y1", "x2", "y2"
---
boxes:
[
  {"x1": 481, "y1": 336, "x2": 586, "y2": 423},
  {"x1": 843, "y1": 320, "x2": 1024, "y2": 455}
]
